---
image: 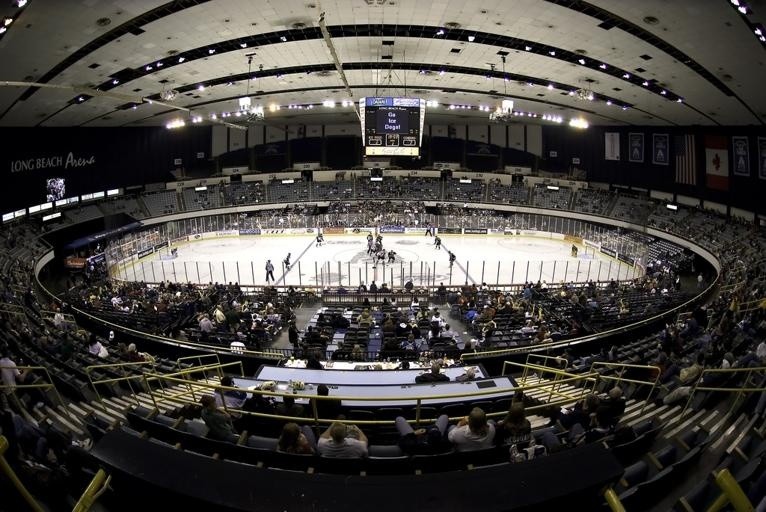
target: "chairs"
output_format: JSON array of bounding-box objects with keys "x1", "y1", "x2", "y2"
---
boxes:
[
  {"x1": 186, "y1": 174, "x2": 539, "y2": 237},
  {"x1": 2, "y1": 173, "x2": 185, "y2": 240},
  {"x1": 3, "y1": 285, "x2": 765, "y2": 511},
  {"x1": 539, "y1": 174, "x2": 638, "y2": 264},
  {"x1": 638, "y1": 174, "x2": 766, "y2": 285},
  {"x1": 2, "y1": 240, "x2": 167, "y2": 285}
]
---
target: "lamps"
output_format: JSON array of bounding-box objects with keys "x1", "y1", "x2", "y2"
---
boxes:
[
  {"x1": 568, "y1": 49, "x2": 594, "y2": 130},
  {"x1": 486, "y1": 49, "x2": 514, "y2": 121},
  {"x1": 238, "y1": 52, "x2": 255, "y2": 112}
]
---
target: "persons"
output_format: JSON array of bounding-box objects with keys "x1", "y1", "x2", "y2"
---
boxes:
[{"x1": 1, "y1": 176, "x2": 766, "y2": 511}]
